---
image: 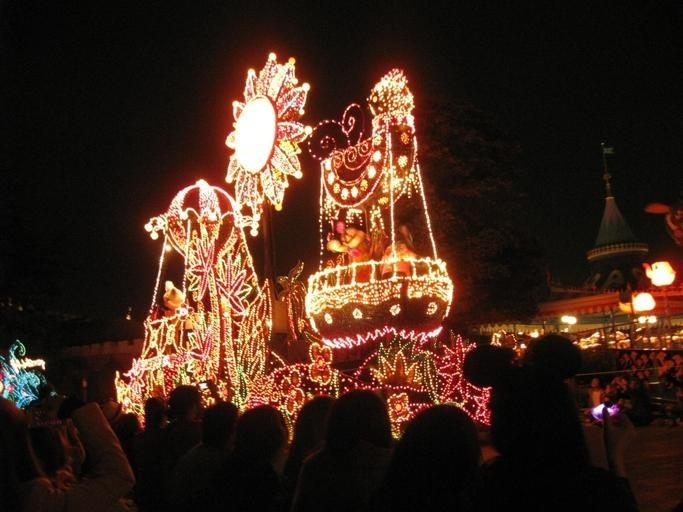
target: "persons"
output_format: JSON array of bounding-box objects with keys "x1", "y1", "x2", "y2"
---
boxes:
[{"x1": 1, "y1": 332, "x2": 683, "y2": 512}]
